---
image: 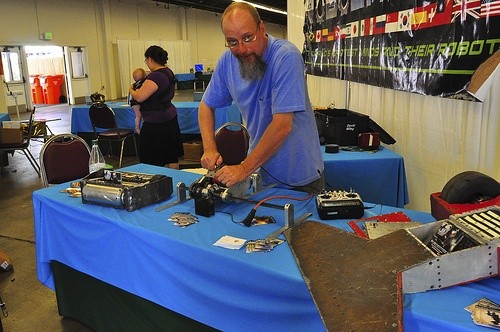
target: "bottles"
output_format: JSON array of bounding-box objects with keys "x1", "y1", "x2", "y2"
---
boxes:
[{"x1": 89, "y1": 140, "x2": 106, "y2": 175}]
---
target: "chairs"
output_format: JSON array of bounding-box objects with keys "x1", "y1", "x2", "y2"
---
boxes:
[
  {"x1": 89, "y1": 102, "x2": 139, "y2": 169},
  {"x1": 0, "y1": 106, "x2": 41, "y2": 178},
  {"x1": 40, "y1": 135, "x2": 92, "y2": 188},
  {"x1": 193, "y1": 71, "x2": 205, "y2": 90},
  {"x1": 215, "y1": 122, "x2": 250, "y2": 165},
  {"x1": 0, "y1": 114, "x2": 10, "y2": 127}
]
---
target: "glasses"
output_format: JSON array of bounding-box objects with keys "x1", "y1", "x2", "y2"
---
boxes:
[
  {"x1": 144, "y1": 57, "x2": 149, "y2": 64},
  {"x1": 225, "y1": 20, "x2": 261, "y2": 48}
]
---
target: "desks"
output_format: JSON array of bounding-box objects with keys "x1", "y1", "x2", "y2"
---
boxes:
[
  {"x1": 320, "y1": 144, "x2": 409, "y2": 209},
  {"x1": 34, "y1": 162, "x2": 500, "y2": 332},
  {"x1": 175, "y1": 73, "x2": 212, "y2": 91},
  {"x1": 70, "y1": 101, "x2": 242, "y2": 157}
]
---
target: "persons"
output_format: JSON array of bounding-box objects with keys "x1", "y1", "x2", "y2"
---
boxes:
[
  {"x1": 198, "y1": 2, "x2": 325, "y2": 194},
  {"x1": 130, "y1": 46, "x2": 184, "y2": 170},
  {"x1": 129, "y1": 68, "x2": 146, "y2": 135}
]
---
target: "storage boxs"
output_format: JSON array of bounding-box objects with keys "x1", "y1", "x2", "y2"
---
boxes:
[
  {"x1": 313, "y1": 109, "x2": 396, "y2": 146},
  {"x1": 183, "y1": 141, "x2": 204, "y2": 161},
  {"x1": 0, "y1": 121, "x2": 24, "y2": 143}
]
